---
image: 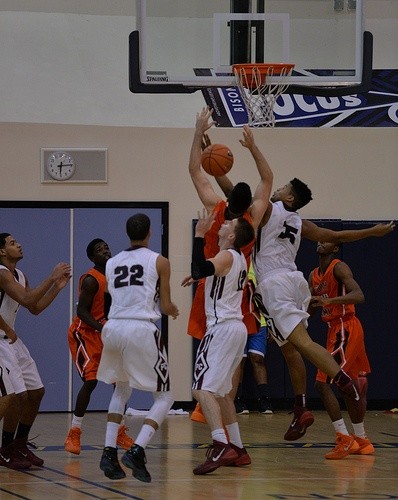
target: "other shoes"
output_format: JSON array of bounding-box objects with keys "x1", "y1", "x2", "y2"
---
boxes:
[
  {"x1": 235, "y1": 403, "x2": 250, "y2": 415},
  {"x1": 254, "y1": 400, "x2": 273, "y2": 414}
]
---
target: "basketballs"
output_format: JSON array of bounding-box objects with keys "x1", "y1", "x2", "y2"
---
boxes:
[{"x1": 201, "y1": 144, "x2": 234, "y2": 176}]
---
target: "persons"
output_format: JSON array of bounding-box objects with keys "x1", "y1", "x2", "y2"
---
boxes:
[
  {"x1": 0, "y1": 233, "x2": 72, "y2": 468},
  {"x1": 192, "y1": 204, "x2": 253, "y2": 474},
  {"x1": 0, "y1": 314, "x2": 17, "y2": 344},
  {"x1": 200, "y1": 133, "x2": 394, "y2": 442},
  {"x1": 189, "y1": 106, "x2": 273, "y2": 422},
  {"x1": 95, "y1": 213, "x2": 181, "y2": 482},
  {"x1": 307, "y1": 239, "x2": 374, "y2": 459},
  {"x1": 64, "y1": 238, "x2": 134, "y2": 454},
  {"x1": 180, "y1": 221, "x2": 298, "y2": 422}
]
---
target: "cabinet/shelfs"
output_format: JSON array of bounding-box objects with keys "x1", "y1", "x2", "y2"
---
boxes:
[{"x1": 192, "y1": 220, "x2": 398, "y2": 411}]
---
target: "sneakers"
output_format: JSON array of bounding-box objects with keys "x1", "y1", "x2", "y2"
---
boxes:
[
  {"x1": 100, "y1": 447, "x2": 126, "y2": 480},
  {"x1": 330, "y1": 369, "x2": 353, "y2": 392},
  {"x1": 121, "y1": 443, "x2": 152, "y2": 482},
  {"x1": 324, "y1": 431, "x2": 354, "y2": 458},
  {"x1": 350, "y1": 434, "x2": 376, "y2": 454},
  {"x1": 116, "y1": 424, "x2": 135, "y2": 450},
  {"x1": 13, "y1": 440, "x2": 43, "y2": 466},
  {"x1": 0, "y1": 447, "x2": 32, "y2": 469},
  {"x1": 193, "y1": 439, "x2": 252, "y2": 474},
  {"x1": 64, "y1": 427, "x2": 82, "y2": 456},
  {"x1": 283, "y1": 405, "x2": 315, "y2": 441}
]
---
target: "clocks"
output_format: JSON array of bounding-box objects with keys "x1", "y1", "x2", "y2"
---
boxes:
[{"x1": 48, "y1": 153, "x2": 76, "y2": 181}]
